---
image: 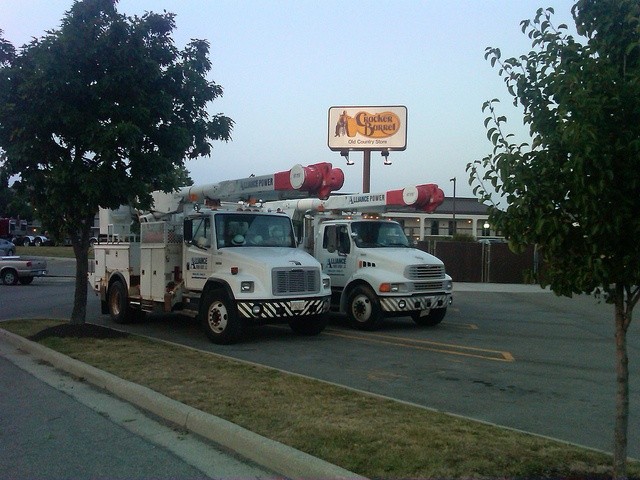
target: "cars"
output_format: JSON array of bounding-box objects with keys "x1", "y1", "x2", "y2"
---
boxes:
[{"x1": 0, "y1": 239, "x2": 17, "y2": 256}]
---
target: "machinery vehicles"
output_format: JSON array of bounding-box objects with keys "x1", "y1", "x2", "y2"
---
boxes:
[
  {"x1": 86, "y1": 162, "x2": 344, "y2": 345},
  {"x1": 259, "y1": 184, "x2": 455, "y2": 332}
]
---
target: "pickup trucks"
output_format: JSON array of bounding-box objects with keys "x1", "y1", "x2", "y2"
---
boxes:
[{"x1": 0, "y1": 255, "x2": 48, "y2": 286}]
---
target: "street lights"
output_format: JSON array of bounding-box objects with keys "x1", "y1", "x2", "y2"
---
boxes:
[{"x1": 484, "y1": 221, "x2": 491, "y2": 236}]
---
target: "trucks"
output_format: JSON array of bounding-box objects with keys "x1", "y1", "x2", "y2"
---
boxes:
[{"x1": 0, "y1": 214, "x2": 55, "y2": 247}]
